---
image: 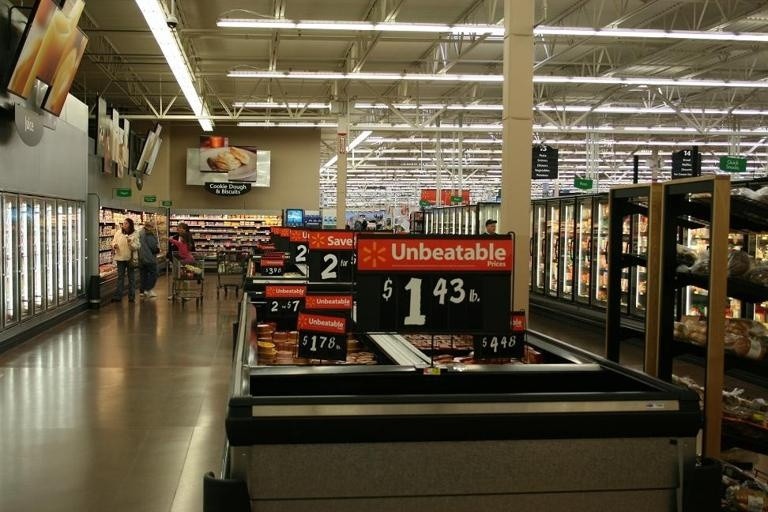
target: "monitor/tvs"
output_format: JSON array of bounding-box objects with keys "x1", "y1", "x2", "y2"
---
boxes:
[{"x1": 282, "y1": 208, "x2": 305, "y2": 228}]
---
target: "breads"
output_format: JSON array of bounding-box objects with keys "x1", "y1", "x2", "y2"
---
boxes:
[{"x1": 206, "y1": 147, "x2": 249, "y2": 171}]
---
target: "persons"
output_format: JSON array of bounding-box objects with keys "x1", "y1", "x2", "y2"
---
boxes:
[
  {"x1": 137, "y1": 220, "x2": 161, "y2": 298},
  {"x1": 159, "y1": 232, "x2": 206, "y2": 284},
  {"x1": 347, "y1": 214, "x2": 406, "y2": 231},
  {"x1": 165, "y1": 223, "x2": 196, "y2": 302},
  {"x1": 110, "y1": 217, "x2": 140, "y2": 302},
  {"x1": 481, "y1": 219, "x2": 497, "y2": 235}
]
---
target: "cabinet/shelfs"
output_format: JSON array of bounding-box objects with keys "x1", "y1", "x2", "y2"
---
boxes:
[{"x1": 168, "y1": 206, "x2": 322, "y2": 273}]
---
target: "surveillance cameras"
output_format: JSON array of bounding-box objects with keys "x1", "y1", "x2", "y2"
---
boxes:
[{"x1": 166, "y1": 16, "x2": 178, "y2": 28}]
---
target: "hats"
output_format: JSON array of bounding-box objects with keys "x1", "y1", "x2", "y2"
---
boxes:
[{"x1": 486, "y1": 219, "x2": 498, "y2": 226}]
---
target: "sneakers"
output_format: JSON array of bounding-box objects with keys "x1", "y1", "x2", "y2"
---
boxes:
[{"x1": 110, "y1": 291, "x2": 190, "y2": 303}]
